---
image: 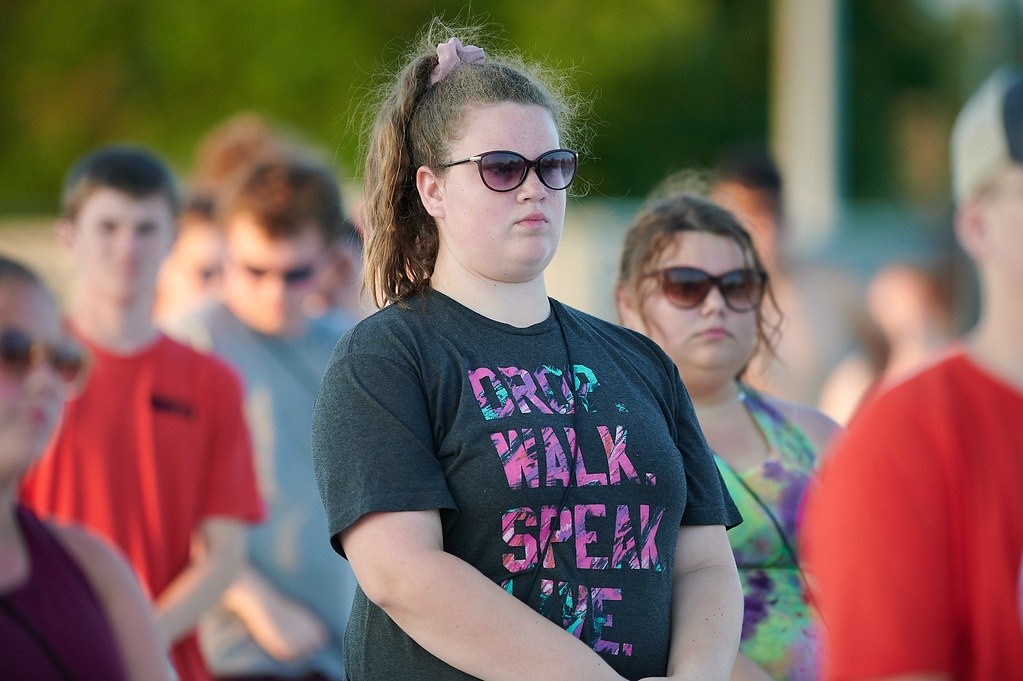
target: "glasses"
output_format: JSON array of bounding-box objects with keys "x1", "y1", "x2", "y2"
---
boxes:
[
  {"x1": 436, "y1": 148, "x2": 580, "y2": 192},
  {"x1": 0, "y1": 322, "x2": 84, "y2": 382},
  {"x1": 638, "y1": 266, "x2": 771, "y2": 314}
]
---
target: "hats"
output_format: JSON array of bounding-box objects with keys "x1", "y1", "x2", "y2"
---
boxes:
[{"x1": 950, "y1": 68, "x2": 1023, "y2": 200}]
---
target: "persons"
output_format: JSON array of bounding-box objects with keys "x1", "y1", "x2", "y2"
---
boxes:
[
  {"x1": 149, "y1": 203, "x2": 227, "y2": 315},
  {"x1": 616, "y1": 198, "x2": 844, "y2": 681},
  {"x1": 822, "y1": 253, "x2": 964, "y2": 429},
  {"x1": 18, "y1": 151, "x2": 268, "y2": 681},
  {"x1": 705, "y1": 161, "x2": 857, "y2": 409},
  {"x1": 163, "y1": 153, "x2": 371, "y2": 681},
  {"x1": 194, "y1": 117, "x2": 287, "y2": 202},
  {"x1": 0, "y1": 255, "x2": 182, "y2": 681},
  {"x1": 800, "y1": 63, "x2": 1023, "y2": 681},
  {"x1": 312, "y1": 35, "x2": 745, "y2": 680},
  {"x1": 314, "y1": 224, "x2": 374, "y2": 320}
]
